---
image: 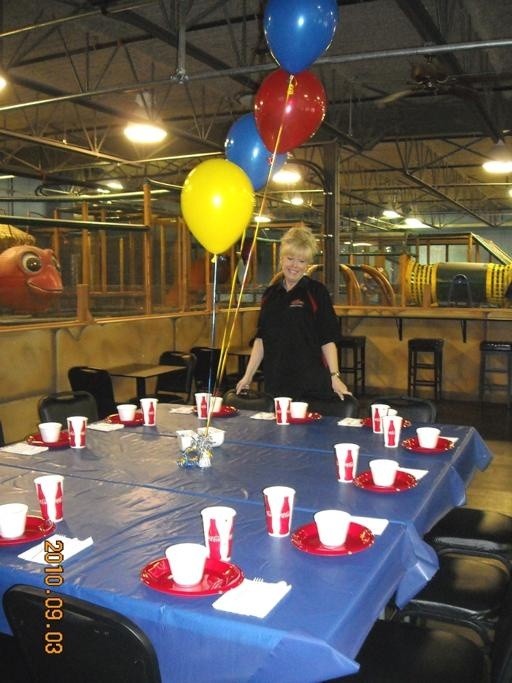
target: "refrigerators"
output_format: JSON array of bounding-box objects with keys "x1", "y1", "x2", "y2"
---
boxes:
[{"x1": 117, "y1": 404, "x2": 138, "y2": 421}]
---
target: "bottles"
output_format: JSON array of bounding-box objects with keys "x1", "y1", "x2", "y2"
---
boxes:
[
  {"x1": 56, "y1": 481, "x2": 64, "y2": 519},
  {"x1": 80, "y1": 420, "x2": 86, "y2": 446},
  {"x1": 69, "y1": 420, "x2": 76, "y2": 446},
  {"x1": 336, "y1": 454, "x2": 341, "y2": 479},
  {"x1": 344, "y1": 449, "x2": 353, "y2": 480},
  {"x1": 285, "y1": 400, "x2": 290, "y2": 422},
  {"x1": 374, "y1": 408, "x2": 381, "y2": 432},
  {"x1": 209, "y1": 518, "x2": 222, "y2": 560},
  {"x1": 265, "y1": 495, "x2": 274, "y2": 532},
  {"x1": 227, "y1": 526, "x2": 233, "y2": 557},
  {"x1": 149, "y1": 402, "x2": 155, "y2": 424},
  {"x1": 201, "y1": 396, "x2": 208, "y2": 418},
  {"x1": 276, "y1": 399, "x2": 283, "y2": 423},
  {"x1": 280, "y1": 496, "x2": 291, "y2": 534},
  {"x1": 387, "y1": 419, "x2": 396, "y2": 445},
  {"x1": 38, "y1": 483, "x2": 49, "y2": 521}
]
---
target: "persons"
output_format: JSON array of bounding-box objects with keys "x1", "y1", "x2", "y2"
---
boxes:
[{"x1": 234, "y1": 223, "x2": 354, "y2": 402}]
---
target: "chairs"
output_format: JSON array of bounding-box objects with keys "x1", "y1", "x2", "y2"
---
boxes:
[
  {"x1": 1, "y1": 581, "x2": 163, "y2": 682},
  {"x1": 36, "y1": 337, "x2": 265, "y2": 434}
]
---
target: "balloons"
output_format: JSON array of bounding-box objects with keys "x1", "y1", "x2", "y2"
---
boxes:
[
  {"x1": 223, "y1": 111, "x2": 286, "y2": 193},
  {"x1": 179, "y1": 158, "x2": 256, "y2": 265},
  {"x1": 262, "y1": 0, "x2": 339, "y2": 75},
  {"x1": 253, "y1": 67, "x2": 326, "y2": 155}
]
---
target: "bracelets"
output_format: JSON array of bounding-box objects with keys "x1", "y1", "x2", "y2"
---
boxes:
[{"x1": 331, "y1": 370, "x2": 341, "y2": 376}]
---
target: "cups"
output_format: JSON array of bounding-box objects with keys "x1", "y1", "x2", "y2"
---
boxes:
[
  {"x1": 370, "y1": 459, "x2": 399, "y2": 486},
  {"x1": 290, "y1": 402, "x2": 308, "y2": 418},
  {"x1": 273, "y1": 397, "x2": 293, "y2": 425},
  {"x1": 201, "y1": 505, "x2": 238, "y2": 561},
  {"x1": 67, "y1": 416, "x2": 89, "y2": 448},
  {"x1": 416, "y1": 426, "x2": 441, "y2": 448},
  {"x1": 176, "y1": 427, "x2": 225, "y2": 448},
  {"x1": 0, "y1": 502, "x2": 28, "y2": 539},
  {"x1": 371, "y1": 404, "x2": 404, "y2": 449},
  {"x1": 195, "y1": 391, "x2": 223, "y2": 420},
  {"x1": 314, "y1": 508, "x2": 350, "y2": 547},
  {"x1": 39, "y1": 421, "x2": 63, "y2": 443},
  {"x1": 140, "y1": 397, "x2": 158, "y2": 426},
  {"x1": 332, "y1": 443, "x2": 361, "y2": 484},
  {"x1": 263, "y1": 484, "x2": 298, "y2": 539},
  {"x1": 164, "y1": 541, "x2": 210, "y2": 586},
  {"x1": 35, "y1": 474, "x2": 65, "y2": 522}
]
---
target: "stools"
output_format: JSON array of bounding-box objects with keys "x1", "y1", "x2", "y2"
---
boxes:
[
  {"x1": 321, "y1": 617, "x2": 488, "y2": 683},
  {"x1": 427, "y1": 505, "x2": 512, "y2": 560},
  {"x1": 478, "y1": 340, "x2": 512, "y2": 407},
  {"x1": 336, "y1": 334, "x2": 366, "y2": 396},
  {"x1": 407, "y1": 337, "x2": 443, "y2": 398},
  {"x1": 385, "y1": 545, "x2": 511, "y2": 683}
]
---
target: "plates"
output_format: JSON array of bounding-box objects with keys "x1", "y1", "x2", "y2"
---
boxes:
[
  {"x1": 24, "y1": 432, "x2": 69, "y2": 447},
  {"x1": 361, "y1": 417, "x2": 372, "y2": 427},
  {"x1": 141, "y1": 558, "x2": 246, "y2": 596},
  {"x1": 192, "y1": 406, "x2": 239, "y2": 416},
  {"x1": 1, "y1": 515, "x2": 58, "y2": 547},
  {"x1": 354, "y1": 470, "x2": 416, "y2": 491},
  {"x1": 106, "y1": 413, "x2": 144, "y2": 425},
  {"x1": 291, "y1": 521, "x2": 376, "y2": 555},
  {"x1": 290, "y1": 411, "x2": 321, "y2": 423},
  {"x1": 402, "y1": 437, "x2": 455, "y2": 453}
]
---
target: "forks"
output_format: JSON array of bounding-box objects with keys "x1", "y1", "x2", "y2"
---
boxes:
[{"x1": 248, "y1": 577, "x2": 264, "y2": 594}]
